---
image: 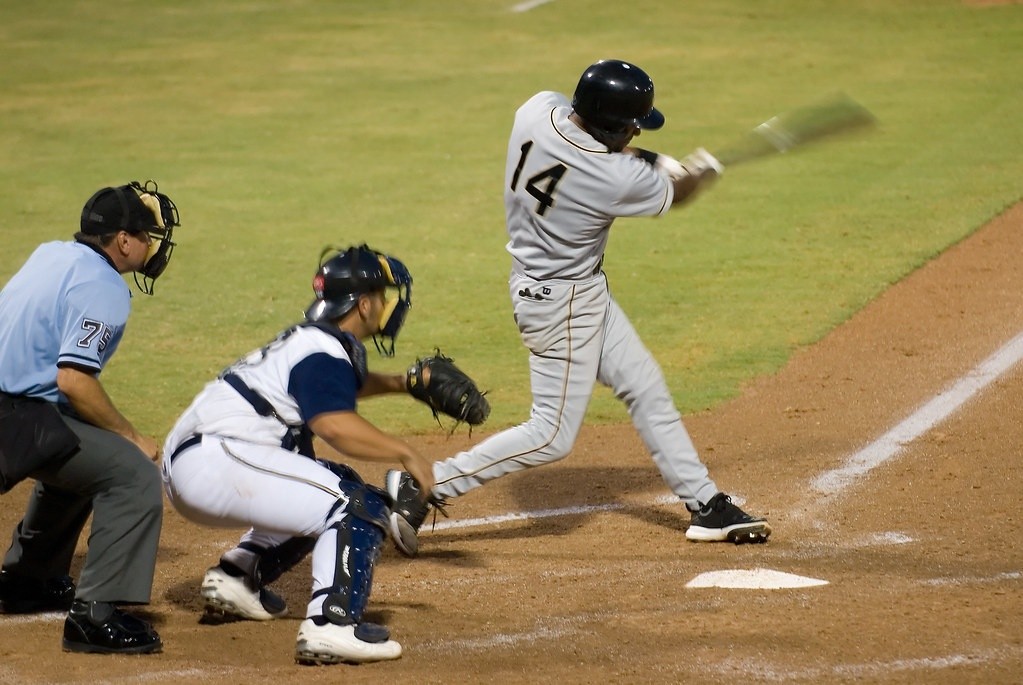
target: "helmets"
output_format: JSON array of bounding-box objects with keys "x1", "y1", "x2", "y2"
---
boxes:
[
  {"x1": 571, "y1": 58, "x2": 665, "y2": 134},
  {"x1": 304, "y1": 250, "x2": 386, "y2": 321}
]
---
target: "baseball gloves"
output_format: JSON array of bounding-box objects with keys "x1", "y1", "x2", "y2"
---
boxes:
[{"x1": 405, "y1": 346, "x2": 492, "y2": 440}]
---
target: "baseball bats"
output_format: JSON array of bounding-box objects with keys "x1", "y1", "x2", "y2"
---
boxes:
[{"x1": 712, "y1": 90, "x2": 876, "y2": 165}]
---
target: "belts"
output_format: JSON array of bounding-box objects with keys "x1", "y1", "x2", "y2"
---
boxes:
[
  {"x1": 169, "y1": 436, "x2": 201, "y2": 462},
  {"x1": 593, "y1": 254, "x2": 604, "y2": 274}
]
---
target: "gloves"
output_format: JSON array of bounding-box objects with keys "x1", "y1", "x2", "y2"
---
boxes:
[
  {"x1": 645, "y1": 146, "x2": 724, "y2": 179},
  {"x1": 519, "y1": 288, "x2": 542, "y2": 299}
]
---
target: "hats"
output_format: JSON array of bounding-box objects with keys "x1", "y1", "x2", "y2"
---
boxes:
[{"x1": 80, "y1": 186, "x2": 158, "y2": 237}]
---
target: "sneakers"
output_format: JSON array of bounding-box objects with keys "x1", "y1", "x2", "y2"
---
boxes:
[
  {"x1": 200, "y1": 567, "x2": 290, "y2": 621},
  {"x1": 387, "y1": 468, "x2": 452, "y2": 557},
  {"x1": 294, "y1": 617, "x2": 403, "y2": 665},
  {"x1": 685, "y1": 491, "x2": 773, "y2": 543}
]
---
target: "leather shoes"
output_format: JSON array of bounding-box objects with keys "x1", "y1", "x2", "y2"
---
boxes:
[
  {"x1": 62, "y1": 602, "x2": 162, "y2": 652},
  {"x1": 0, "y1": 569, "x2": 77, "y2": 613}
]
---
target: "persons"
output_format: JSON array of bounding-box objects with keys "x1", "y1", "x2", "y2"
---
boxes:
[
  {"x1": 161, "y1": 242, "x2": 490, "y2": 665},
  {"x1": 0, "y1": 181, "x2": 180, "y2": 656},
  {"x1": 384, "y1": 59, "x2": 772, "y2": 559}
]
